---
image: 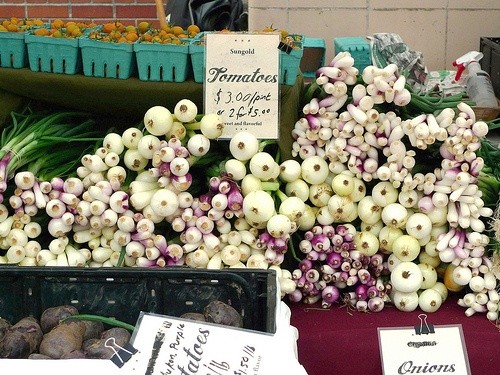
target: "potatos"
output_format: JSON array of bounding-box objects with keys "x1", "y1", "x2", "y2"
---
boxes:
[{"x1": 0, "y1": 300, "x2": 245, "y2": 360}]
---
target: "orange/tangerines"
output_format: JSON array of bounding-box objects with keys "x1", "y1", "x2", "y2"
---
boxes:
[{"x1": 0, "y1": 16, "x2": 302, "y2": 80}]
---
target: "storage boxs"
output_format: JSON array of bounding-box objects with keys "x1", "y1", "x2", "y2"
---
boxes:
[
  {"x1": 479, "y1": 36, "x2": 500, "y2": 101},
  {"x1": 188, "y1": 32, "x2": 208, "y2": 84},
  {"x1": 300, "y1": 37, "x2": 326, "y2": 77},
  {"x1": 0, "y1": 26, "x2": 38, "y2": 68},
  {"x1": 278, "y1": 34, "x2": 303, "y2": 86},
  {"x1": 333, "y1": 36, "x2": 372, "y2": 78},
  {"x1": 79, "y1": 26, "x2": 138, "y2": 79},
  {"x1": 24, "y1": 26, "x2": 85, "y2": 76},
  {"x1": 134, "y1": 28, "x2": 196, "y2": 82}
]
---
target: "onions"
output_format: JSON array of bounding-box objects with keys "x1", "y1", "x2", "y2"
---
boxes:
[{"x1": 0, "y1": 52, "x2": 500, "y2": 321}]
